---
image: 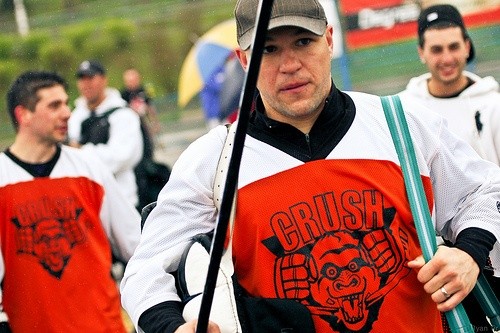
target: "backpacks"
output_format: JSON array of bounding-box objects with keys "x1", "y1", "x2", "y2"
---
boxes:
[{"x1": 80, "y1": 108, "x2": 122, "y2": 144}]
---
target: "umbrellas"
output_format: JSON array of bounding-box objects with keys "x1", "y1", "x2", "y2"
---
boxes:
[{"x1": 175, "y1": 15, "x2": 245, "y2": 108}]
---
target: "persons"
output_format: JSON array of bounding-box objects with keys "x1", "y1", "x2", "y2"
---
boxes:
[
  {"x1": 65, "y1": 61, "x2": 143, "y2": 211},
  {"x1": 0, "y1": 70, "x2": 144, "y2": 333},
  {"x1": 119, "y1": 67, "x2": 163, "y2": 163},
  {"x1": 199, "y1": 56, "x2": 231, "y2": 133},
  {"x1": 118, "y1": 1, "x2": 499, "y2": 333},
  {"x1": 394, "y1": 4, "x2": 500, "y2": 277}
]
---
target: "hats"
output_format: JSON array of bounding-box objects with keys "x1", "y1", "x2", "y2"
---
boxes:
[
  {"x1": 75, "y1": 60, "x2": 105, "y2": 76},
  {"x1": 234, "y1": 0, "x2": 327, "y2": 51},
  {"x1": 418, "y1": 5, "x2": 475, "y2": 63}
]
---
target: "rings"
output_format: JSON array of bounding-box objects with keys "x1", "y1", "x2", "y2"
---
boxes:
[{"x1": 440, "y1": 287, "x2": 450, "y2": 298}]
